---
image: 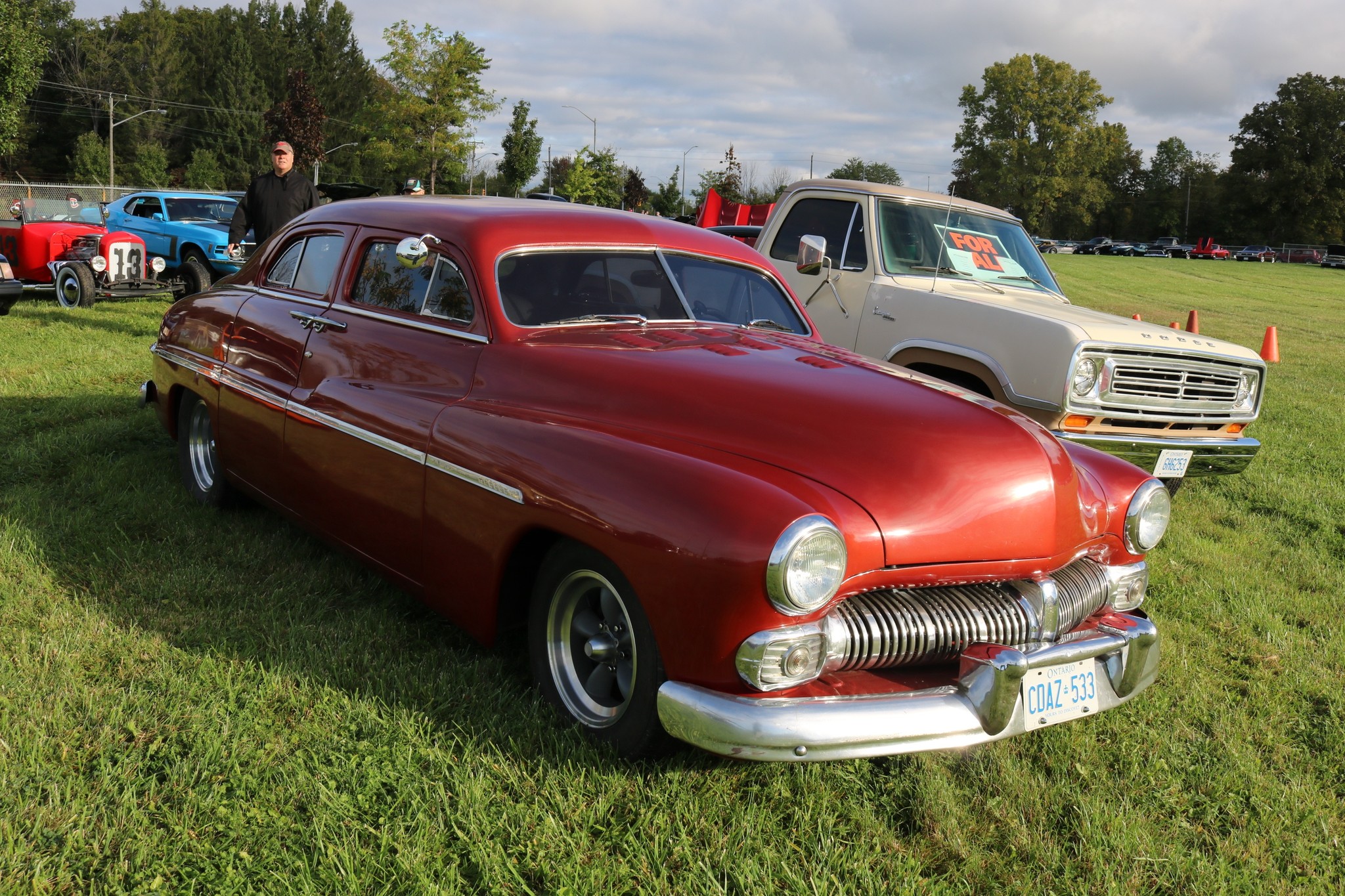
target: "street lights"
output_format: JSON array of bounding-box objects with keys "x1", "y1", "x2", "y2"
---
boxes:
[
  {"x1": 682, "y1": 146, "x2": 699, "y2": 216},
  {"x1": 109, "y1": 99, "x2": 167, "y2": 203},
  {"x1": 469, "y1": 153, "x2": 499, "y2": 195},
  {"x1": 649, "y1": 176, "x2": 667, "y2": 191},
  {"x1": 863, "y1": 161, "x2": 874, "y2": 182},
  {"x1": 562, "y1": 105, "x2": 596, "y2": 154},
  {"x1": 314, "y1": 143, "x2": 357, "y2": 186}
]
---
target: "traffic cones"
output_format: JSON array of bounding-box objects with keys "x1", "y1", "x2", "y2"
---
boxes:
[
  {"x1": 1186, "y1": 310, "x2": 1198, "y2": 335},
  {"x1": 1132, "y1": 314, "x2": 1141, "y2": 322},
  {"x1": 1259, "y1": 326, "x2": 1279, "y2": 363},
  {"x1": 1169, "y1": 322, "x2": 1180, "y2": 330}
]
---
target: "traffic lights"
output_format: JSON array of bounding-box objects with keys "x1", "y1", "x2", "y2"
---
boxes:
[{"x1": 482, "y1": 189, "x2": 485, "y2": 196}]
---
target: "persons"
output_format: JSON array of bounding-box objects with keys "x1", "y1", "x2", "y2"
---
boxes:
[
  {"x1": 628, "y1": 208, "x2": 661, "y2": 217},
  {"x1": 227, "y1": 141, "x2": 320, "y2": 258},
  {"x1": 402, "y1": 178, "x2": 426, "y2": 196}
]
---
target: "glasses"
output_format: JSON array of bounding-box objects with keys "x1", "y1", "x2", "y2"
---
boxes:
[{"x1": 405, "y1": 189, "x2": 422, "y2": 192}]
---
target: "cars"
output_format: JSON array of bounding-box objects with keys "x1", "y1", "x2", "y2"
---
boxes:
[
  {"x1": 1320, "y1": 244, "x2": 1345, "y2": 267},
  {"x1": 137, "y1": 196, "x2": 1167, "y2": 761},
  {"x1": 1189, "y1": 238, "x2": 1230, "y2": 260},
  {"x1": 0, "y1": 191, "x2": 258, "y2": 318},
  {"x1": 1275, "y1": 249, "x2": 1322, "y2": 264},
  {"x1": 1109, "y1": 243, "x2": 1148, "y2": 256},
  {"x1": 1236, "y1": 245, "x2": 1277, "y2": 263},
  {"x1": 1031, "y1": 236, "x2": 1080, "y2": 254}
]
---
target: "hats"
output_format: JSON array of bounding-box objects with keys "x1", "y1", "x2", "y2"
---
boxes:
[
  {"x1": 402, "y1": 177, "x2": 424, "y2": 192},
  {"x1": 271, "y1": 141, "x2": 294, "y2": 155}
]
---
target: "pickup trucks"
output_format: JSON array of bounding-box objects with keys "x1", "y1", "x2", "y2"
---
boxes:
[
  {"x1": 1144, "y1": 237, "x2": 1193, "y2": 258},
  {"x1": 707, "y1": 179, "x2": 1266, "y2": 497},
  {"x1": 1077, "y1": 237, "x2": 1120, "y2": 255}
]
